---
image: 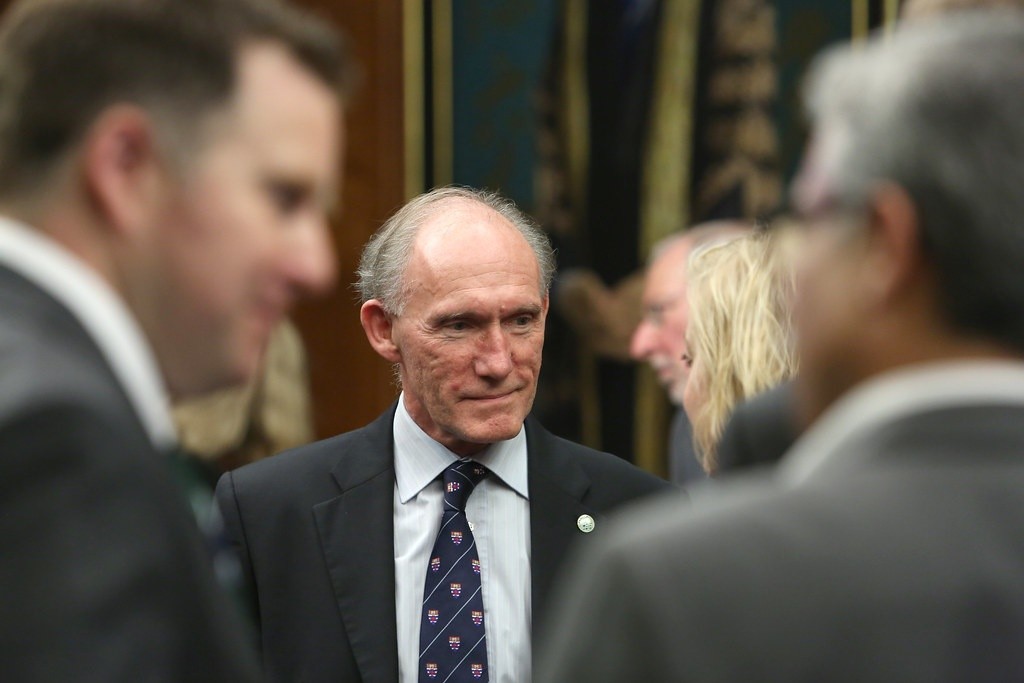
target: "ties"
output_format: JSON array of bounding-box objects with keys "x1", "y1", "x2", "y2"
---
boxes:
[{"x1": 418, "y1": 461, "x2": 489, "y2": 683}]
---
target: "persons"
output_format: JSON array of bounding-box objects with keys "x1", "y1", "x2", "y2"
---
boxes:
[
  {"x1": 535, "y1": 1, "x2": 1024, "y2": 682},
  {"x1": 216, "y1": 186, "x2": 683, "y2": 682},
  {"x1": 0, "y1": 0, "x2": 339, "y2": 682}
]
